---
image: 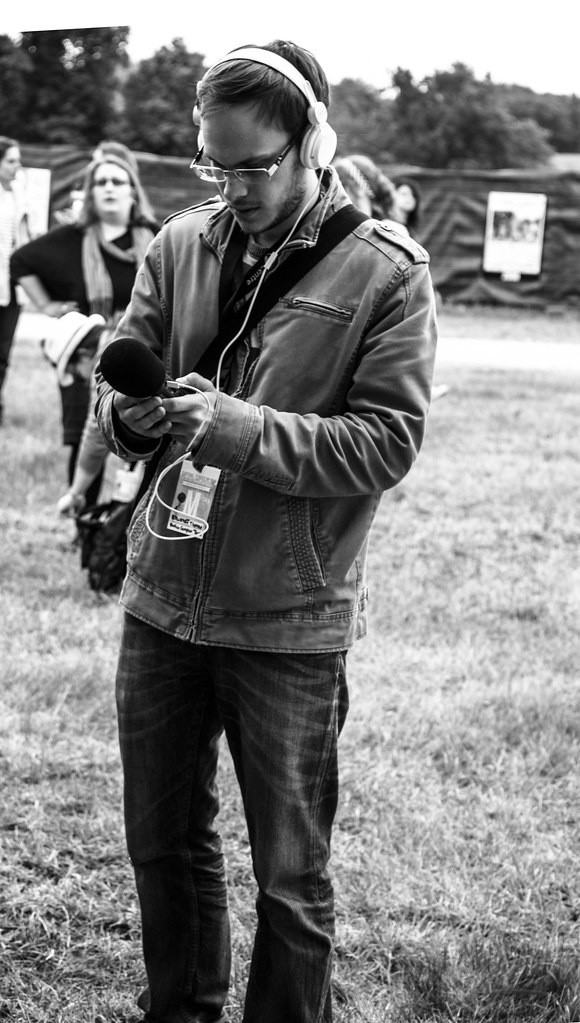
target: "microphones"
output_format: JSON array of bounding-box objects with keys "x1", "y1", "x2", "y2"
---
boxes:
[{"x1": 100, "y1": 338, "x2": 187, "y2": 400}]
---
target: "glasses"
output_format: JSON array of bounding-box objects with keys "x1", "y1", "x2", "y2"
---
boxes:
[{"x1": 189, "y1": 140, "x2": 299, "y2": 182}]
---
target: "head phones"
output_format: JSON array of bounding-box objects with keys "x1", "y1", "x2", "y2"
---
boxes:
[{"x1": 197, "y1": 48, "x2": 337, "y2": 170}]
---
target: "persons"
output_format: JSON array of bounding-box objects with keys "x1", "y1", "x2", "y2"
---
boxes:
[
  {"x1": 329, "y1": 152, "x2": 408, "y2": 227},
  {"x1": 0, "y1": 130, "x2": 40, "y2": 423},
  {"x1": 85, "y1": 34, "x2": 442, "y2": 1023},
  {"x1": 77, "y1": 140, "x2": 143, "y2": 222},
  {"x1": 48, "y1": 308, "x2": 145, "y2": 597},
  {"x1": 371, "y1": 174, "x2": 419, "y2": 233},
  {"x1": 8, "y1": 154, "x2": 169, "y2": 527}
]
